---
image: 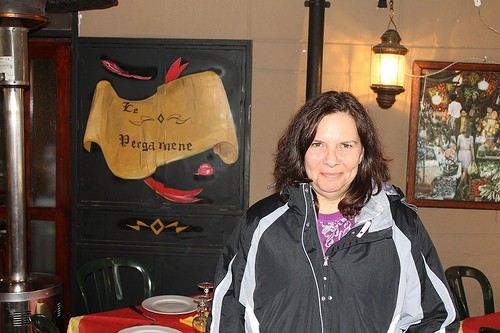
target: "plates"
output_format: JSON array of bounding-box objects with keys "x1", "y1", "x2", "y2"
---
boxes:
[
  {"x1": 118, "y1": 325, "x2": 183, "y2": 333},
  {"x1": 142, "y1": 295, "x2": 200, "y2": 314}
]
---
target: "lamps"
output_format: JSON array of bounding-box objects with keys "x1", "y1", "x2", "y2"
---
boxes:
[{"x1": 369, "y1": 0, "x2": 409, "y2": 110}]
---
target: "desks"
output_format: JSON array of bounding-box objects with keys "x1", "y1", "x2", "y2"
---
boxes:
[
  {"x1": 458, "y1": 311, "x2": 500, "y2": 333},
  {"x1": 66, "y1": 290, "x2": 215, "y2": 333}
]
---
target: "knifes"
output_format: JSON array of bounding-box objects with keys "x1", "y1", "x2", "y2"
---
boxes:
[{"x1": 128, "y1": 304, "x2": 157, "y2": 321}]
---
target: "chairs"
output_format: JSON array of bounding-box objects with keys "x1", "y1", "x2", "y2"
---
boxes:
[
  {"x1": 75, "y1": 258, "x2": 157, "y2": 315},
  {"x1": 446, "y1": 266, "x2": 495, "y2": 320}
]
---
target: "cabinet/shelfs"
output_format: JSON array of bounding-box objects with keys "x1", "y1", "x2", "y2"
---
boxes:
[{"x1": 72, "y1": 34, "x2": 251, "y2": 315}]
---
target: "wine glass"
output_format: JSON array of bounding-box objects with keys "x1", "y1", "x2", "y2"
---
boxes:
[
  {"x1": 197, "y1": 282, "x2": 215, "y2": 317},
  {"x1": 192, "y1": 296, "x2": 212, "y2": 333}
]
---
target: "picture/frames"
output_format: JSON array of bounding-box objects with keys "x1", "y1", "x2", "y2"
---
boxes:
[{"x1": 405, "y1": 61, "x2": 500, "y2": 210}]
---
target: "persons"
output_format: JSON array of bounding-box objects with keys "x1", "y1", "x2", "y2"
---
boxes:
[{"x1": 207, "y1": 91, "x2": 460, "y2": 333}]
